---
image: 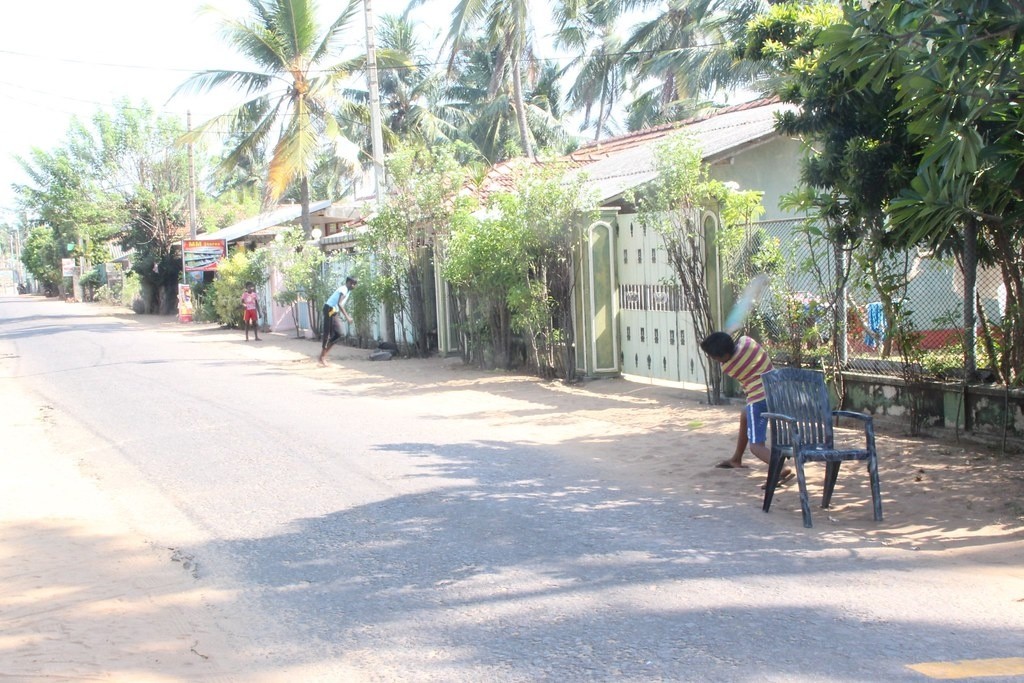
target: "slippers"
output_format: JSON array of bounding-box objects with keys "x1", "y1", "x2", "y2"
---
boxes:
[
  {"x1": 715, "y1": 460, "x2": 749, "y2": 469},
  {"x1": 761, "y1": 473, "x2": 796, "y2": 490}
]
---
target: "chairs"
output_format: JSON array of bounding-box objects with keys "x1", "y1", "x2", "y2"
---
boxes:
[{"x1": 761, "y1": 367, "x2": 882, "y2": 527}]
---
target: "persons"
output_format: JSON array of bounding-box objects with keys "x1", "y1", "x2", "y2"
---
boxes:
[
  {"x1": 241, "y1": 281, "x2": 263, "y2": 341},
  {"x1": 319, "y1": 276, "x2": 357, "y2": 366},
  {"x1": 700, "y1": 331, "x2": 796, "y2": 490},
  {"x1": 997, "y1": 274, "x2": 1024, "y2": 324}
]
